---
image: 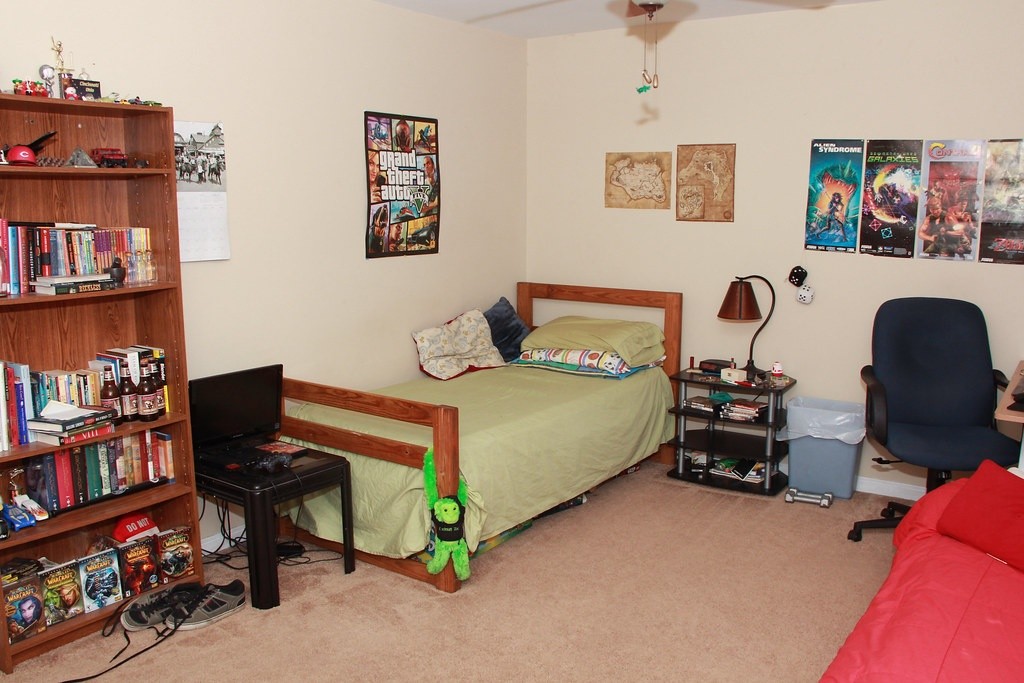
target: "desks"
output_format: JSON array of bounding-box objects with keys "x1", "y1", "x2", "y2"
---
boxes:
[{"x1": 194, "y1": 438, "x2": 354, "y2": 610}]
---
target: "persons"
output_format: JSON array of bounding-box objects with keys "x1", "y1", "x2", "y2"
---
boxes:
[
  {"x1": 39, "y1": 65, "x2": 54, "y2": 98},
  {"x1": 50, "y1": 36, "x2": 64, "y2": 68},
  {"x1": 64, "y1": 87, "x2": 78, "y2": 101},
  {"x1": 174, "y1": 149, "x2": 223, "y2": 183}
]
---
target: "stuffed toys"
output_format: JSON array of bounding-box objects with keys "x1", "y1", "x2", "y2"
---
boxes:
[{"x1": 422, "y1": 447, "x2": 471, "y2": 581}]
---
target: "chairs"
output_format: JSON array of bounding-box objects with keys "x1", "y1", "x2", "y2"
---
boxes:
[{"x1": 846, "y1": 298, "x2": 1021, "y2": 543}]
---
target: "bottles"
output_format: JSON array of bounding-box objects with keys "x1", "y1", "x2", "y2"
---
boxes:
[
  {"x1": 134, "y1": 250, "x2": 146, "y2": 284},
  {"x1": 101, "y1": 365, "x2": 123, "y2": 427},
  {"x1": 147, "y1": 357, "x2": 166, "y2": 416},
  {"x1": 145, "y1": 250, "x2": 157, "y2": 282},
  {"x1": 136, "y1": 362, "x2": 158, "y2": 422},
  {"x1": 117, "y1": 362, "x2": 139, "y2": 422},
  {"x1": 122, "y1": 251, "x2": 139, "y2": 287}
]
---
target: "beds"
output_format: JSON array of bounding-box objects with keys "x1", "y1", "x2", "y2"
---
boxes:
[
  {"x1": 273, "y1": 281, "x2": 682, "y2": 593},
  {"x1": 819, "y1": 468, "x2": 1024, "y2": 681}
]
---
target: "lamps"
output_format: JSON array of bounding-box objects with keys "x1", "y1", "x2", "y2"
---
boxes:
[
  {"x1": 632, "y1": 0, "x2": 667, "y2": 89},
  {"x1": 716, "y1": 275, "x2": 775, "y2": 379}
]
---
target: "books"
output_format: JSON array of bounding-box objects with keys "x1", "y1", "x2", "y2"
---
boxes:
[
  {"x1": 718, "y1": 398, "x2": 769, "y2": 422},
  {"x1": 683, "y1": 395, "x2": 724, "y2": 413},
  {"x1": 0, "y1": 218, "x2": 153, "y2": 296},
  {"x1": 0, "y1": 524, "x2": 195, "y2": 646},
  {"x1": 25, "y1": 429, "x2": 177, "y2": 518},
  {"x1": 0, "y1": 344, "x2": 170, "y2": 452}
]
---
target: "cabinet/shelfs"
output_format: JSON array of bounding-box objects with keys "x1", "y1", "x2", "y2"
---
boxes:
[
  {"x1": 0, "y1": 92, "x2": 203, "y2": 671},
  {"x1": 669, "y1": 368, "x2": 796, "y2": 496}
]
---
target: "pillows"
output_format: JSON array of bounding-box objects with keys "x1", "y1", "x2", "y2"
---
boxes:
[
  {"x1": 484, "y1": 297, "x2": 533, "y2": 361},
  {"x1": 412, "y1": 310, "x2": 507, "y2": 379},
  {"x1": 937, "y1": 459, "x2": 1024, "y2": 573}
]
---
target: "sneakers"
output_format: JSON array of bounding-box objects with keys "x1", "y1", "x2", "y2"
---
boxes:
[
  {"x1": 165, "y1": 579, "x2": 244, "y2": 630},
  {"x1": 121, "y1": 581, "x2": 202, "y2": 630}
]
